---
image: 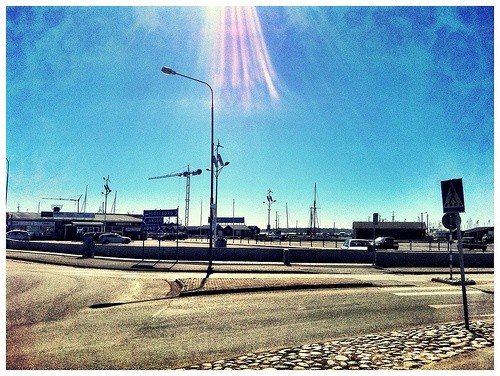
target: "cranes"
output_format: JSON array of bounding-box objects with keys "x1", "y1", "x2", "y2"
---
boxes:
[
  {"x1": 149, "y1": 166, "x2": 202, "y2": 229},
  {"x1": 40, "y1": 193, "x2": 83, "y2": 213}
]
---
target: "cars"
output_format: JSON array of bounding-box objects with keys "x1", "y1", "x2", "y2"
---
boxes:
[
  {"x1": 455, "y1": 237, "x2": 487, "y2": 251},
  {"x1": 82, "y1": 231, "x2": 132, "y2": 244},
  {"x1": 42, "y1": 230, "x2": 57, "y2": 240},
  {"x1": 433, "y1": 237, "x2": 447, "y2": 242},
  {"x1": 157, "y1": 232, "x2": 189, "y2": 241},
  {"x1": 6, "y1": 229, "x2": 31, "y2": 241},
  {"x1": 340, "y1": 237, "x2": 399, "y2": 251}
]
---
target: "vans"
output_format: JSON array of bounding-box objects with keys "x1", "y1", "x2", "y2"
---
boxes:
[{"x1": 254, "y1": 232, "x2": 276, "y2": 242}]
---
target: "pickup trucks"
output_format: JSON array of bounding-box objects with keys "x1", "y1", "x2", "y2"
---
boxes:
[{"x1": 334, "y1": 232, "x2": 351, "y2": 238}]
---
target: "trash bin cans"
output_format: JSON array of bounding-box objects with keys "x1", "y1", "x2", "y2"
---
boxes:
[{"x1": 82, "y1": 234, "x2": 96, "y2": 259}]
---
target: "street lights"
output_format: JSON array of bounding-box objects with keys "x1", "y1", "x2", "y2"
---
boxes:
[
  {"x1": 102, "y1": 175, "x2": 111, "y2": 224},
  {"x1": 207, "y1": 140, "x2": 230, "y2": 248},
  {"x1": 161, "y1": 66, "x2": 214, "y2": 274},
  {"x1": 262, "y1": 188, "x2": 277, "y2": 233}
]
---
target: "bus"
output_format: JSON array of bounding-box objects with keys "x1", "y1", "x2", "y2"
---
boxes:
[{"x1": 435, "y1": 232, "x2": 448, "y2": 237}]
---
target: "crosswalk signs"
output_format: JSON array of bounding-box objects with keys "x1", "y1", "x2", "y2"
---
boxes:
[{"x1": 441, "y1": 178, "x2": 465, "y2": 213}]
